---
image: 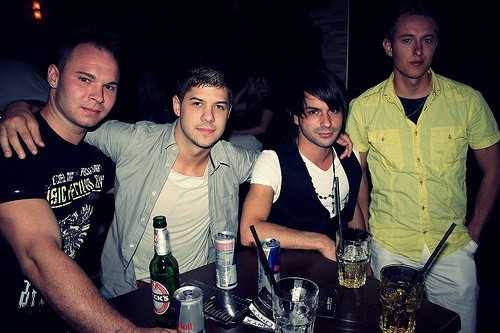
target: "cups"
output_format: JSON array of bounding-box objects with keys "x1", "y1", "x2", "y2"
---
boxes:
[
  {"x1": 335, "y1": 227, "x2": 373, "y2": 288},
  {"x1": 271, "y1": 277, "x2": 319, "y2": 333},
  {"x1": 378, "y1": 263, "x2": 427, "y2": 333}
]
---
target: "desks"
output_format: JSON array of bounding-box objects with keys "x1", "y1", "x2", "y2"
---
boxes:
[{"x1": 42, "y1": 247, "x2": 462, "y2": 333}]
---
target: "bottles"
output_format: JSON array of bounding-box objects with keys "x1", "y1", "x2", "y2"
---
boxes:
[{"x1": 149, "y1": 215, "x2": 179, "y2": 330}]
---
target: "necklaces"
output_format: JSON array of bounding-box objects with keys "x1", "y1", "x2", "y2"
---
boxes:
[
  {"x1": 404, "y1": 84, "x2": 432, "y2": 118},
  {"x1": 300, "y1": 148, "x2": 335, "y2": 212}
]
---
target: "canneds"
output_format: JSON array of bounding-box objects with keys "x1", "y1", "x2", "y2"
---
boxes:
[
  {"x1": 213, "y1": 230, "x2": 238, "y2": 289},
  {"x1": 173, "y1": 286, "x2": 206, "y2": 333},
  {"x1": 257, "y1": 238, "x2": 281, "y2": 297}
]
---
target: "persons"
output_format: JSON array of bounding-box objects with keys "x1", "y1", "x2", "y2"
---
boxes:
[
  {"x1": 0, "y1": 38, "x2": 179, "y2": 333},
  {"x1": 240, "y1": 72, "x2": 364, "y2": 262},
  {"x1": 344, "y1": 7, "x2": 500, "y2": 333},
  {"x1": 0, "y1": 68, "x2": 352, "y2": 298}
]
